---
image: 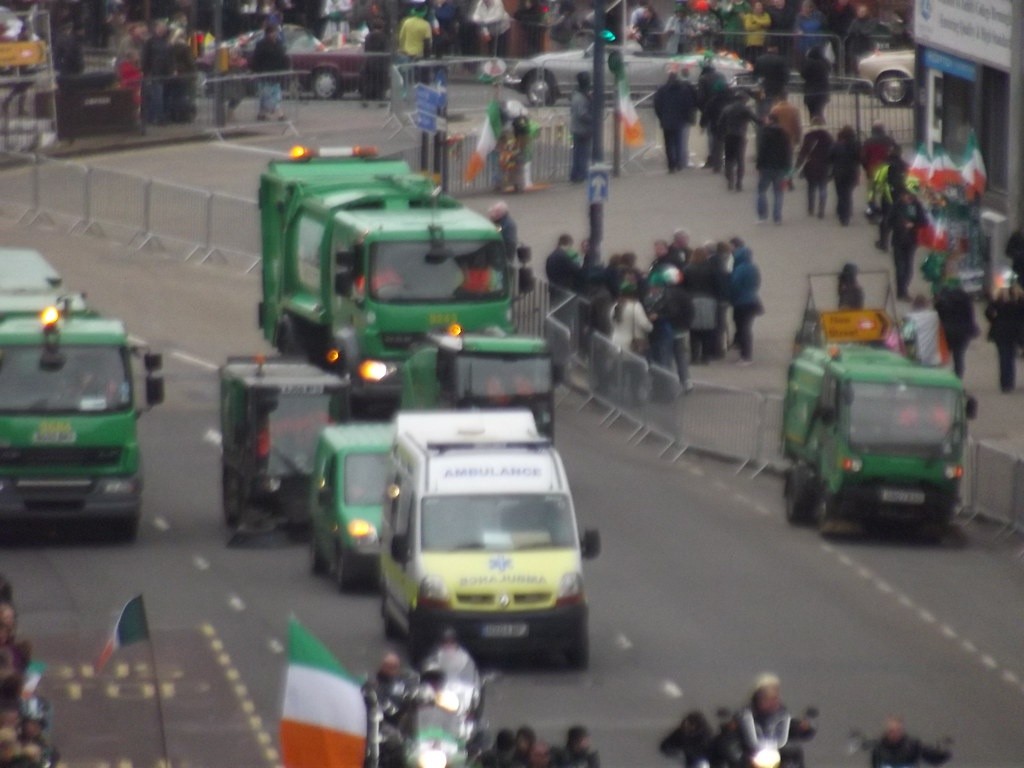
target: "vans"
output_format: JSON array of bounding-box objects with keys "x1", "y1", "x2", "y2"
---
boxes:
[{"x1": 308, "y1": 422, "x2": 397, "y2": 595}]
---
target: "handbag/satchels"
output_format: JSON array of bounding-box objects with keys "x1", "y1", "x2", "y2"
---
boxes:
[
  {"x1": 632, "y1": 337, "x2": 648, "y2": 354},
  {"x1": 796, "y1": 155, "x2": 811, "y2": 179},
  {"x1": 745, "y1": 300, "x2": 766, "y2": 316}
]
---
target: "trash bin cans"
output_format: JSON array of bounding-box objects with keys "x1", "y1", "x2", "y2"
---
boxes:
[{"x1": 980, "y1": 212, "x2": 1008, "y2": 272}]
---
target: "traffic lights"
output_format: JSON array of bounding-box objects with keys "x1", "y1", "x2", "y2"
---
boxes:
[{"x1": 600, "y1": 0, "x2": 626, "y2": 48}]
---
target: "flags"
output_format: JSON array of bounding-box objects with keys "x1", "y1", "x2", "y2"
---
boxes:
[
  {"x1": 281, "y1": 618, "x2": 368, "y2": 768},
  {"x1": 466, "y1": 100, "x2": 502, "y2": 180},
  {"x1": 619, "y1": 80, "x2": 644, "y2": 146},
  {"x1": 914, "y1": 134, "x2": 986, "y2": 194},
  {"x1": 96, "y1": 596, "x2": 150, "y2": 675}
]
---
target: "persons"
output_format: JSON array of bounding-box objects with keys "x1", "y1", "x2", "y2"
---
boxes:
[
  {"x1": 661, "y1": 676, "x2": 815, "y2": 768},
  {"x1": 0, "y1": 0, "x2": 212, "y2": 124},
  {"x1": 838, "y1": 191, "x2": 1024, "y2": 393},
  {"x1": 571, "y1": 73, "x2": 594, "y2": 180},
  {"x1": 872, "y1": 718, "x2": 921, "y2": 768},
  {"x1": 0, "y1": 573, "x2": 59, "y2": 768},
  {"x1": 374, "y1": 628, "x2": 600, "y2": 768},
  {"x1": 222, "y1": 0, "x2": 575, "y2": 121},
  {"x1": 630, "y1": 0, "x2": 911, "y2": 72},
  {"x1": 546, "y1": 229, "x2": 763, "y2": 407},
  {"x1": 653, "y1": 46, "x2": 908, "y2": 248},
  {"x1": 491, "y1": 200, "x2": 518, "y2": 263}
]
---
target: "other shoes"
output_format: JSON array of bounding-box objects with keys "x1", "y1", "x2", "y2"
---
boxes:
[
  {"x1": 727, "y1": 342, "x2": 743, "y2": 352},
  {"x1": 684, "y1": 382, "x2": 694, "y2": 393},
  {"x1": 736, "y1": 358, "x2": 753, "y2": 366},
  {"x1": 876, "y1": 241, "x2": 888, "y2": 250},
  {"x1": 898, "y1": 294, "x2": 913, "y2": 303}
]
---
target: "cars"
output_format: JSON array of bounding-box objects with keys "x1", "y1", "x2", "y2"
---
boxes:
[
  {"x1": 855, "y1": 45, "x2": 917, "y2": 109},
  {"x1": 0, "y1": 0, "x2": 197, "y2": 138}
]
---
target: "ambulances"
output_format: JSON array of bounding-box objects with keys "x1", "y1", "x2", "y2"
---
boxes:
[{"x1": 375, "y1": 409, "x2": 603, "y2": 675}]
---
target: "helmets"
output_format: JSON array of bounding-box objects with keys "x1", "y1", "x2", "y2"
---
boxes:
[
  {"x1": 489, "y1": 201, "x2": 508, "y2": 223},
  {"x1": 755, "y1": 675, "x2": 779, "y2": 694},
  {"x1": 664, "y1": 269, "x2": 683, "y2": 286}
]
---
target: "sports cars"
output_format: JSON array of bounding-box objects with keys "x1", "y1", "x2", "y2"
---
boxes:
[
  {"x1": 504, "y1": 34, "x2": 762, "y2": 109},
  {"x1": 192, "y1": 22, "x2": 394, "y2": 111}
]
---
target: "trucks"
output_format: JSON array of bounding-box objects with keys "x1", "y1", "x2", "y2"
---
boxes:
[
  {"x1": 217, "y1": 356, "x2": 353, "y2": 548},
  {"x1": 0, "y1": 246, "x2": 167, "y2": 544},
  {"x1": 396, "y1": 330, "x2": 569, "y2": 447},
  {"x1": 779, "y1": 342, "x2": 980, "y2": 545},
  {"x1": 256, "y1": 144, "x2": 536, "y2": 421}
]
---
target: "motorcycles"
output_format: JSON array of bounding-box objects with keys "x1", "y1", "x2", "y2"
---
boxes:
[{"x1": 359, "y1": 670, "x2": 500, "y2": 767}]
